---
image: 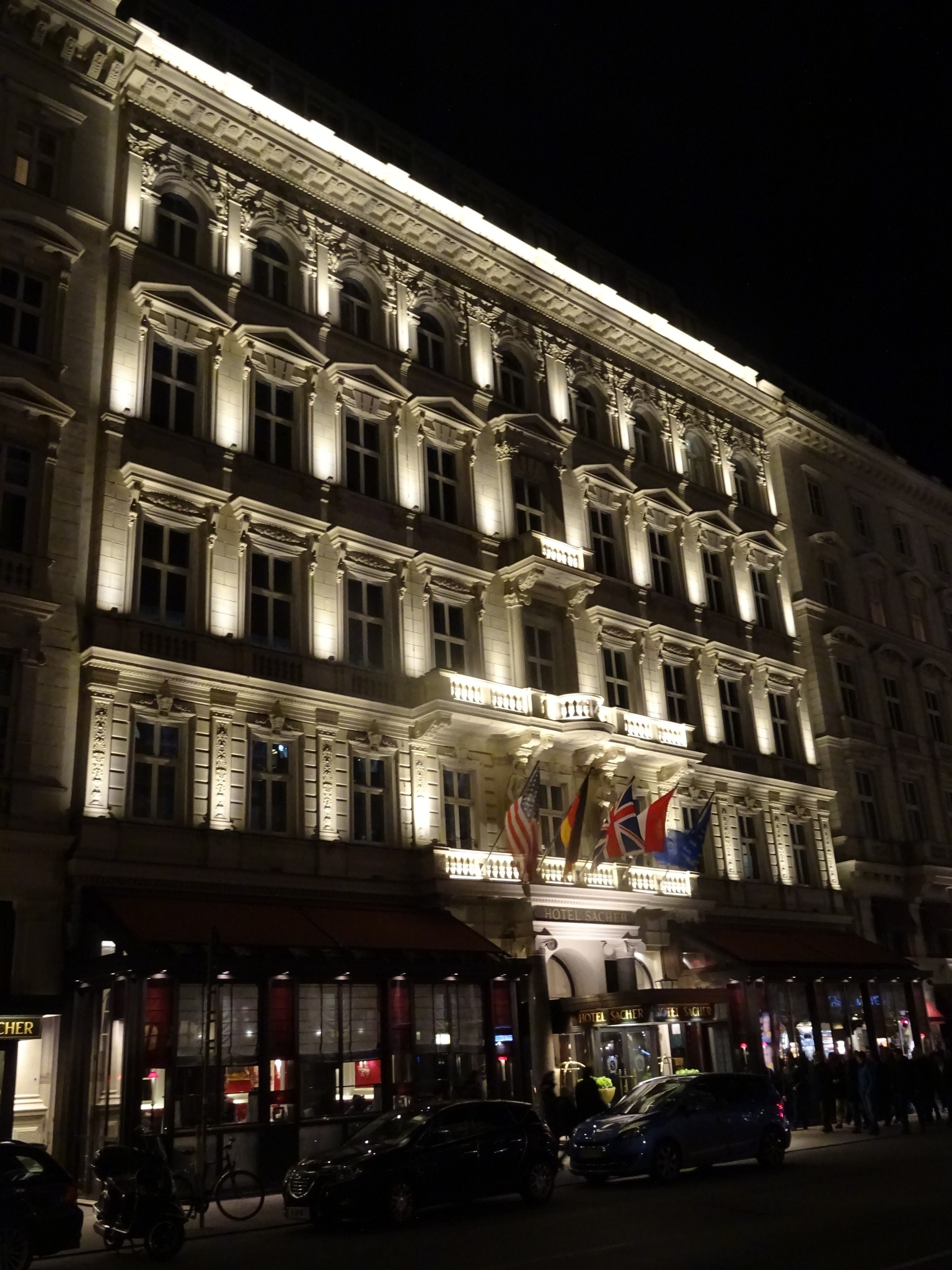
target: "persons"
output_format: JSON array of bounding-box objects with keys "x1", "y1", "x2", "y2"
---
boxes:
[
  {"x1": 538, "y1": 1066, "x2": 607, "y2": 1150},
  {"x1": 769, "y1": 1039, "x2": 952, "y2": 1136}
]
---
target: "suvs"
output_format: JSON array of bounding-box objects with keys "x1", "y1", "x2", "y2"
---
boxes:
[
  {"x1": 567, "y1": 1070, "x2": 793, "y2": 1189},
  {"x1": 281, "y1": 1099, "x2": 561, "y2": 1239}
]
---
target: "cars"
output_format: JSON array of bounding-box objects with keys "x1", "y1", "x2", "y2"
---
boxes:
[{"x1": 0, "y1": 1138, "x2": 83, "y2": 1270}]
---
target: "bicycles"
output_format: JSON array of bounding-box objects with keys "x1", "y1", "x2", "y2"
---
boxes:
[{"x1": 170, "y1": 1136, "x2": 268, "y2": 1222}]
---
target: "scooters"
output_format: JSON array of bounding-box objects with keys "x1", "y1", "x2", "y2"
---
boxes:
[{"x1": 93, "y1": 1153, "x2": 187, "y2": 1260}]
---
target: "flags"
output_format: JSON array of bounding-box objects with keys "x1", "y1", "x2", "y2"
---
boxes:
[
  {"x1": 591, "y1": 775, "x2": 644, "y2": 869},
  {"x1": 560, "y1": 766, "x2": 594, "y2": 882},
  {"x1": 653, "y1": 791, "x2": 715, "y2": 870},
  {"x1": 636, "y1": 781, "x2": 681, "y2": 852},
  {"x1": 503, "y1": 760, "x2": 543, "y2": 886}
]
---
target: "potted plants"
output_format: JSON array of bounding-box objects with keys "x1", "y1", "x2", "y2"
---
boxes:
[{"x1": 574, "y1": 1074, "x2": 618, "y2": 1106}]
